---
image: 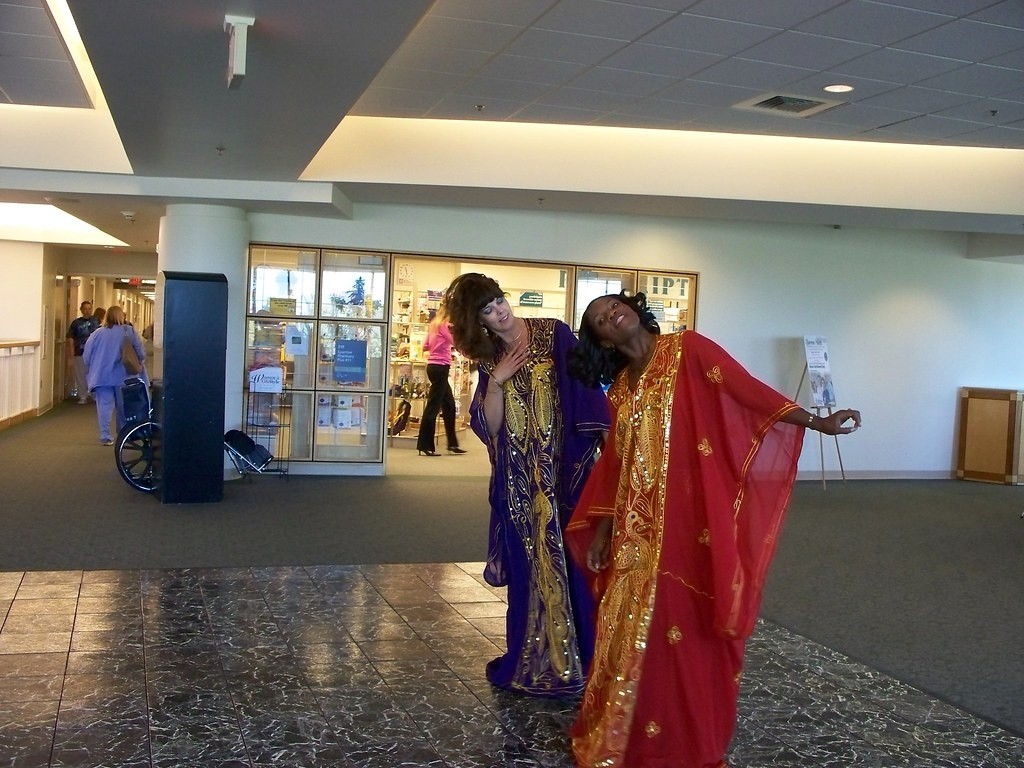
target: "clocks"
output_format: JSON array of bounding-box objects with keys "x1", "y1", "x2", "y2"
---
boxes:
[{"x1": 398, "y1": 263, "x2": 415, "y2": 281}]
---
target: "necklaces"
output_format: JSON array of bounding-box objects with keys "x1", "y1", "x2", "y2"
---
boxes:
[{"x1": 506, "y1": 318, "x2": 523, "y2": 344}]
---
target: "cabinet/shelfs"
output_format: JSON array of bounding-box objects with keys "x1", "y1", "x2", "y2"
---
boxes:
[{"x1": 387, "y1": 285, "x2": 471, "y2": 451}]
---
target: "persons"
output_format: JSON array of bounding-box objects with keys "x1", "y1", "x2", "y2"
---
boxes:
[
  {"x1": 415, "y1": 299, "x2": 467, "y2": 458},
  {"x1": 811, "y1": 375, "x2": 835, "y2": 406},
  {"x1": 563, "y1": 288, "x2": 863, "y2": 768},
  {"x1": 66, "y1": 301, "x2": 154, "y2": 445},
  {"x1": 446, "y1": 273, "x2": 609, "y2": 697},
  {"x1": 251, "y1": 310, "x2": 285, "y2": 414}
]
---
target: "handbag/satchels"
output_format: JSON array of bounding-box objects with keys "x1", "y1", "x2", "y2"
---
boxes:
[{"x1": 122, "y1": 324, "x2": 140, "y2": 374}]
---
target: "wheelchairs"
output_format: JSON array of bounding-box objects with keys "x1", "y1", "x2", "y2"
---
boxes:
[{"x1": 115, "y1": 363, "x2": 274, "y2": 494}]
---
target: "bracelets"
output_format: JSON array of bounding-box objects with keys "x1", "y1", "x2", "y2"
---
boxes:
[
  {"x1": 489, "y1": 372, "x2": 503, "y2": 386},
  {"x1": 809, "y1": 414, "x2": 816, "y2": 429}
]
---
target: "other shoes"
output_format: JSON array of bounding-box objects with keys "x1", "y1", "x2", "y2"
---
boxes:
[
  {"x1": 103, "y1": 441, "x2": 113, "y2": 446},
  {"x1": 78, "y1": 399, "x2": 88, "y2": 404}
]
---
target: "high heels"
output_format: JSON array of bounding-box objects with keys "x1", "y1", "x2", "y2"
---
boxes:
[
  {"x1": 447, "y1": 447, "x2": 467, "y2": 455},
  {"x1": 419, "y1": 450, "x2": 441, "y2": 456}
]
